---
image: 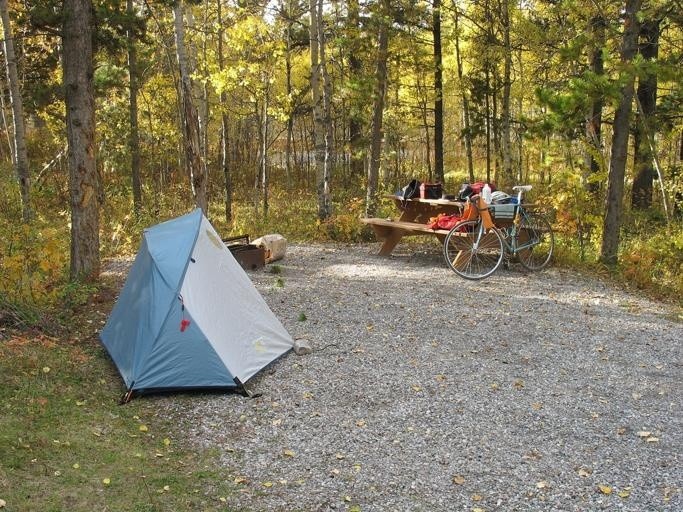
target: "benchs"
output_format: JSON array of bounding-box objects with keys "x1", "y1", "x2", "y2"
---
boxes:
[
  {"x1": 358, "y1": 216, "x2": 494, "y2": 269},
  {"x1": 514, "y1": 223, "x2": 559, "y2": 261}
]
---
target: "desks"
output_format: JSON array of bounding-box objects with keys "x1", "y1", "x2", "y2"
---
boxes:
[{"x1": 380, "y1": 194, "x2": 538, "y2": 269}]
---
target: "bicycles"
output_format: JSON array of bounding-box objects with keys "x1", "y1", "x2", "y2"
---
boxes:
[{"x1": 443, "y1": 185, "x2": 554, "y2": 281}]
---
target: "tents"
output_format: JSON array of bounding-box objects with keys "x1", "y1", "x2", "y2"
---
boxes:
[{"x1": 98, "y1": 208, "x2": 299, "y2": 405}]
